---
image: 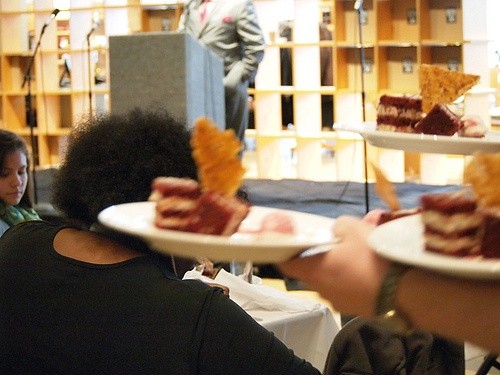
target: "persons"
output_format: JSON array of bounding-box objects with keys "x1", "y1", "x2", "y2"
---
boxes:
[
  {"x1": 0, "y1": 107, "x2": 500, "y2": 375},
  {"x1": 178, "y1": 0, "x2": 265, "y2": 157}
]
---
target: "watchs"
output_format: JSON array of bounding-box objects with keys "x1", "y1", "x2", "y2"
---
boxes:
[{"x1": 374, "y1": 263, "x2": 414, "y2": 334}]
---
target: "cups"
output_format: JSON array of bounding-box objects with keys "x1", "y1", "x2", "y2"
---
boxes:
[{"x1": 230, "y1": 262, "x2": 253, "y2": 284}]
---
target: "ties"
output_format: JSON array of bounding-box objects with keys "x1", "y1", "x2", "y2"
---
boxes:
[{"x1": 199, "y1": 0, "x2": 209, "y2": 25}]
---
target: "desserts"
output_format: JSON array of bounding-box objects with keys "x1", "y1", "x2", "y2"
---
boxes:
[
  {"x1": 375, "y1": 64, "x2": 487, "y2": 139},
  {"x1": 187, "y1": 116, "x2": 252, "y2": 237},
  {"x1": 418, "y1": 152, "x2": 500, "y2": 260},
  {"x1": 152, "y1": 177, "x2": 200, "y2": 229}
]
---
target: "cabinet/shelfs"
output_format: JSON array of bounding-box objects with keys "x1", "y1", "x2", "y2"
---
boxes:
[{"x1": 0, "y1": 0, "x2": 491, "y2": 183}]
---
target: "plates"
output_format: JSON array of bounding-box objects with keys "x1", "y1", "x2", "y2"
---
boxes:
[
  {"x1": 333, "y1": 122, "x2": 500, "y2": 155},
  {"x1": 367, "y1": 212, "x2": 500, "y2": 280},
  {"x1": 252, "y1": 274, "x2": 263, "y2": 285},
  {"x1": 97, "y1": 201, "x2": 343, "y2": 263}
]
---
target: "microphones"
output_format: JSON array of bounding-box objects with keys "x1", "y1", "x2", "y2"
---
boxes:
[
  {"x1": 87, "y1": 22, "x2": 101, "y2": 37},
  {"x1": 45, "y1": 9, "x2": 60, "y2": 26},
  {"x1": 354, "y1": 0, "x2": 364, "y2": 9}
]
